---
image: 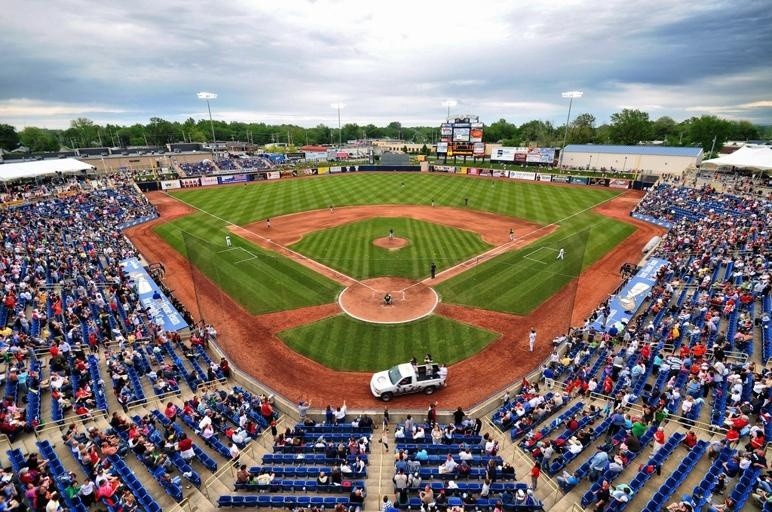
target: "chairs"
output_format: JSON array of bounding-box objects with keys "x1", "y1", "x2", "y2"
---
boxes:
[
  {"x1": 542, "y1": 241, "x2": 772, "y2": 389},
  {"x1": 629, "y1": 181, "x2": 772, "y2": 249},
  {"x1": 224, "y1": 378, "x2": 772, "y2": 512},
  {"x1": 0, "y1": 180, "x2": 228, "y2": 512}
]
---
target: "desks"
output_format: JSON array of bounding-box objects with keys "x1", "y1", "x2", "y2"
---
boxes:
[
  {"x1": 559, "y1": 91, "x2": 584, "y2": 168},
  {"x1": 442, "y1": 101, "x2": 455, "y2": 124},
  {"x1": 197, "y1": 91, "x2": 219, "y2": 161},
  {"x1": 330, "y1": 102, "x2": 346, "y2": 152}
]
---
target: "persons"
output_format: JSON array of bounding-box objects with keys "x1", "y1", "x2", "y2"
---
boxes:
[
  {"x1": 388, "y1": 228, "x2": 395, "y2": 239},
  {"x1": 528, "y1": 326, "x2": 536, "y2": 352},
  {"x1": 264, "y1": 217, "x2": 274, "y2": 233},
  {"x1": 382, "y1": 292, "x2": 394, "y2": 306},
  {"x1": 225, "y1": 234, "x2": 232, "y2": 248},
  {"x1": 327, "y1": 205, "x2": 338, "y2": 217},
  {"x1": 507, "y1": 228, "x2": 514, "y2": 242},
  {"x1": 0, "y1": 384, "x2": 282, "y2": 509},
  {"x1": 3, "y1": 158, "x2": 232, "y2": 442},
  {"x1": 562, "y1": 171, "x2": 772, "y2": 512},
  {"x1": 218, "y1": 349, "x2": 564, "y2": 512},
  {"x1": 429, "y1": 263, "x2": 438, "y2": 280},
  {"x1": 554, "y1": 246, "x2": 568, "y2": 261},
  {"x1": 463, "y1": 197, "x2": 469, "y2": 209}
]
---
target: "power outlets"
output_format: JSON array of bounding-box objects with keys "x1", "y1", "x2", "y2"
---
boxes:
[{"x1": 369, "y1": 362, "x2": 445, "y2": 403}]
